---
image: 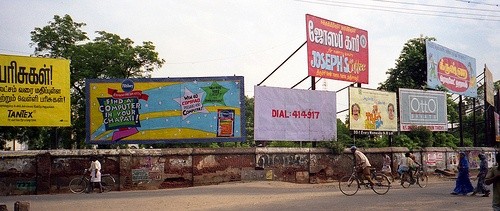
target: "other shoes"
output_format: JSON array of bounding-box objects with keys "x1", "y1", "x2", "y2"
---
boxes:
[{"x1": 450, "y1": 192, "x2": 457, "y2": 195}]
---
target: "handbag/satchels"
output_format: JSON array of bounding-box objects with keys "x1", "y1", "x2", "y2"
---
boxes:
[{"x1": 383, "y1": 165, "x2": 391, "y2": 173}]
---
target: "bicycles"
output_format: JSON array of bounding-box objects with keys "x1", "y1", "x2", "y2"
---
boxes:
[
  {"x1": 338, "y1": 163, "x2": 392, "y2": 196},
  {"x1": 68, "y1": 167, "x2": 116, "y2": 194},
  {"x1": 400, "y1": 164, "x2": 429, "y2": 189}
]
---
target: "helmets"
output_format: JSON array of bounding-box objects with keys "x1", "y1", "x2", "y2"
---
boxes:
[{"x1": 350, "y1": 146, "x2": 357, "y2": 150}]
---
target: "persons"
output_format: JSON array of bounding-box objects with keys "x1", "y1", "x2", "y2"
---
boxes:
[
  {"x1": 484, "y1": 150, "x2": 500, "y2": 211},
  {"x1": 351, "y1": 103, "x2": 365, "y2": 129},
  {"x1": 470, "y1": 153, "x2": 491, "y2": 196},
  {"x1": 86, "y1": 155, "x2": 104, "y2": 194},
  {"x1": 383, "y1": 103, "x2": 396, "y2": 129},
  {"x1": 450, "y1": 151, "x2": 475, "y2": 195},
  {"x1": 397, "y1": 151, "x2": 421, "y2": 185},
  {"x1": 382, "y1": 153, "x2": 394, "y2": 182},
  {"x1": 350, "y1": 146, "x2": 374, "y2": 189}
]
